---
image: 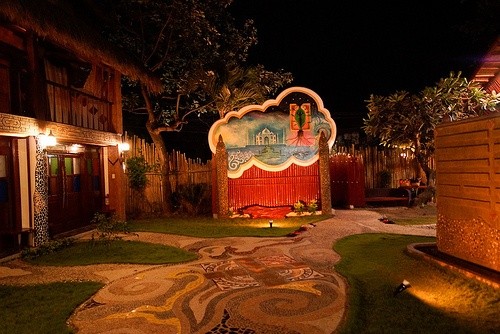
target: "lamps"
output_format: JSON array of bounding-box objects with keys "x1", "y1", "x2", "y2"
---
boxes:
[
  {"x1": 40, "y1": 130, "x2": 58, "y2": 153},
  {"x1": 119, "y1": 142, "x2": 131, "y2": 161}
]
